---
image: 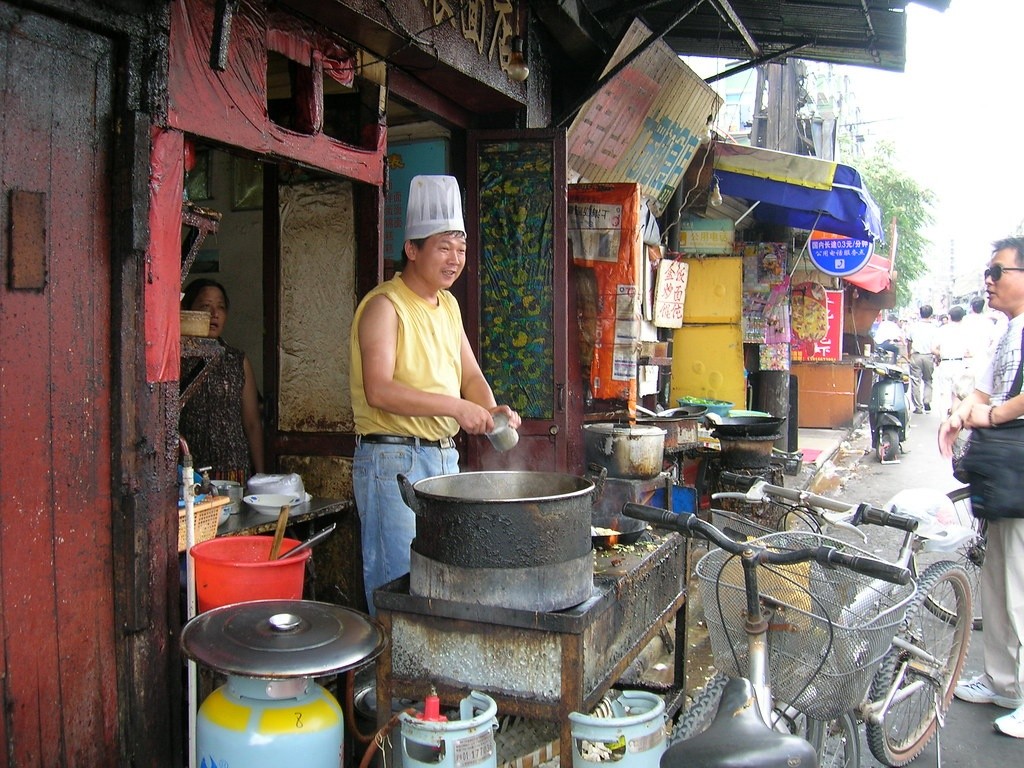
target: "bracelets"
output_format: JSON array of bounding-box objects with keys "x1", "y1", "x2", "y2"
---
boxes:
[
  {"x1": 988, "y1": 404, "x2": 998, "y2": 429},
  {"x1": 955, "y1": 413, "x2": 964, "y2": 431}
]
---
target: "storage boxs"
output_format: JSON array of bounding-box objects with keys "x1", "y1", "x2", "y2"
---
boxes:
[{"x1": 179, "y1": 495, "x2": 231, "y2": 552}]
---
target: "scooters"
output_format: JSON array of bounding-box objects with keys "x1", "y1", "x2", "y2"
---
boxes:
[{"x1": 859, "y1": 346, "x2": 921, "y2": 464}]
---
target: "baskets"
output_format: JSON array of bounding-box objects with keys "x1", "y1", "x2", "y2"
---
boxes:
[
  {"x1": 707, "y1": 508, "x2": 776, "y2": 552},
  {"x1": 177, "y1": 494, "x2": 230, "y2": 552},
  {"x1": 677, "y1": 397, "x2": 733, "y2": 417},
  {"x1": 694, "y1": 530, "x2": 917, "y2": 721}
]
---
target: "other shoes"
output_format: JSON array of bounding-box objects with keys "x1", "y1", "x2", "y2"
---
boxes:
[
  {"x1": 913, "y1": 410, "x2": 922, "y2": 414},
  {"x1": 923, "y1": 402, "x2": 931, "y2": 411}
]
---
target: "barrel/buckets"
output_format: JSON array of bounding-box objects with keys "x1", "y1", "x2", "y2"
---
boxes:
[{"x1": 191, "y1": 535, "x2": 311, "y2": 615}]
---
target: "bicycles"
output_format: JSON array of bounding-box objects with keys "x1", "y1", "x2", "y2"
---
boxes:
[
  {"x1": 905, "y1": 409, "x2": 986, "y2": 632},
  {"x1": 709, "y1": 468, "x2": 974, "y2": 768},
  {"x1": 619, "y1": 500, "x2": 910, "y2": 768}
]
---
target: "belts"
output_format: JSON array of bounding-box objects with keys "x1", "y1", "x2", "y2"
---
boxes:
[
  {"x1": 357, "y1": 433, "x2": 454, "y2": 449},
  {"x1": 942, "y1": 358, "x2": 962, "y2": 361}
]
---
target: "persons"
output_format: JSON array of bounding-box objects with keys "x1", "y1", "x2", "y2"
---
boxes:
[
  {"x1": 938, "y1": 235, "x2": 1024, "y2": 739},
  {"x1": 180, "y1": 278, "x2": 265, "y2": 497},
  {"x1": 874, "y1": 297, "x2": 995, "y2": 427},
  {"x1": 350, "y1": 177, "x2": 521, "y2": 621}
]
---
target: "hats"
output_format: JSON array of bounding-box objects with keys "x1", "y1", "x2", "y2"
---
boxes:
[{"x1": 403, "y1": 175, "x2": 467, "y2": 241}]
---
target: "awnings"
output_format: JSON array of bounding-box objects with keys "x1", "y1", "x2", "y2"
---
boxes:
[
  {"x1": 842, "y1": 252, "x2": 892, "y2": 293},
  {"x1": 548, "y1": 0, "x2": 950, "y2": 130},
  {"x1": 712, "y1": 140, "x2": 884, "y2": 279}
]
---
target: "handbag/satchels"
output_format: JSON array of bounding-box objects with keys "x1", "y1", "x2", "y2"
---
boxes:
[{"x1": 962, "y1": 417, "x2": 1024, "y2": 519}]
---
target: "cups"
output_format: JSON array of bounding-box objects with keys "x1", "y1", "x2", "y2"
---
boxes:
[
  {"x1": 216, "y1": 487, "x2": 246, "y2": 514},
  {"x1": 485, "y1": 413, "x2": 519, "y2": 452}
]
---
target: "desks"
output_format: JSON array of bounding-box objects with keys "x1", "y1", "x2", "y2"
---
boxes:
[{"x1": 367, "y1": 526, "x2": 693, "y2": 768}]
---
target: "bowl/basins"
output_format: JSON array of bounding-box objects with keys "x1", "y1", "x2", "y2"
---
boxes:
[
  {"x1": 675, "y1": 397, "x2": 734, "y2": 423},
  {"x1": 218, "y1": 500, "x2": 236, "y2": 527},
  {"x1": 211, "y1": 479, "x2": 241, "y2": 487},
  {"x1": 244, "y1": 494, "x2": 303, "y2": 515}
]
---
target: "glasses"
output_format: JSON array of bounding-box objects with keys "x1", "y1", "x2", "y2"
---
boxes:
[{"x1": 984, "y1": 265, "x2": 1024, "y2": 281}]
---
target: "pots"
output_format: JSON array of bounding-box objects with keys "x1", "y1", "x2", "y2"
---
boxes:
[
  {"x1": 581, "y1": 416, "x2": 667, "y2": 480},
  {"x1": 707, "y1": 416, "x2": 787, "y2": 437},
  {"x1": 395, "y1": 462, "x2": 610, "y2": 570},
  {"x1": 636, "y1": 405, "x2": 707, "y2": 418}
]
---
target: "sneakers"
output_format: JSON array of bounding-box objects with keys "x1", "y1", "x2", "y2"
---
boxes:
[
  {"x1": 953, "y1": 676, "x2": 1023, "y2": 710},
  {"x1": 993, "y1": 705, "x2": 1024, "y2": 738}
]
